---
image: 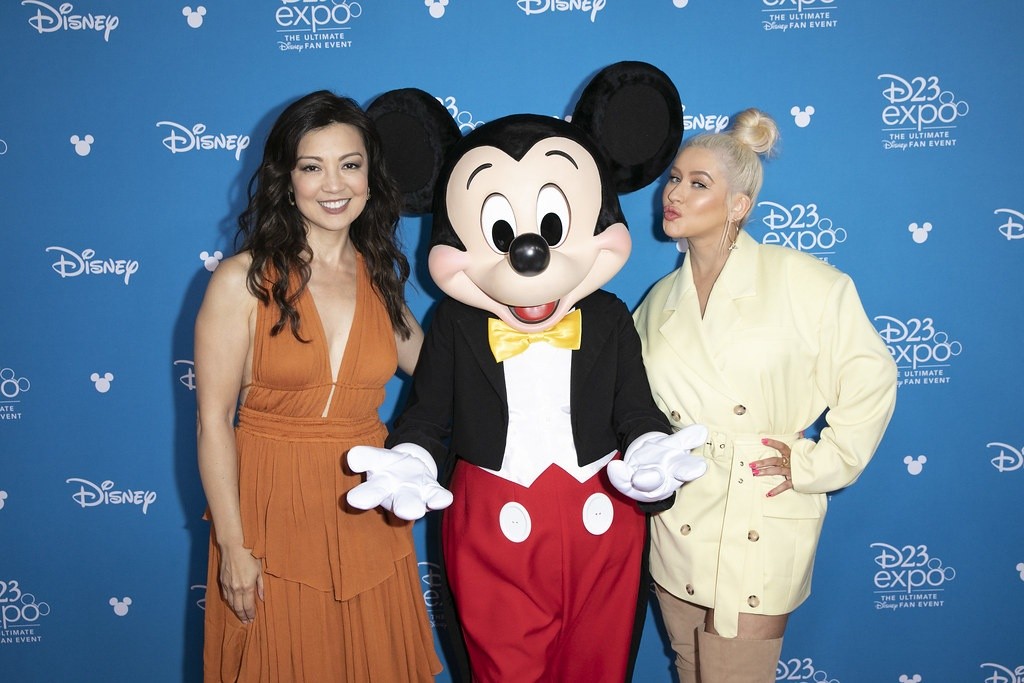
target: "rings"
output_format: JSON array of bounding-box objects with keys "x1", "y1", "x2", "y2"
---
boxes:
[{"x1": 783, "y1": 456, "x2": 789, "y2": 466}]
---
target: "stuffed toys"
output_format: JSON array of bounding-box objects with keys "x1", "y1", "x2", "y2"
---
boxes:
[{"x1": 345, "y1": 59, "x2": 708, "y2": 682}]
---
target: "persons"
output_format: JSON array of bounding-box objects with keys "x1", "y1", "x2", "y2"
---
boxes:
[
  {"x1": 195, "y1": 88, "x2": 443, "y2": 683},
  {"x1": 628, "y1": 106, "x2": 897, "y2": 683}
]
